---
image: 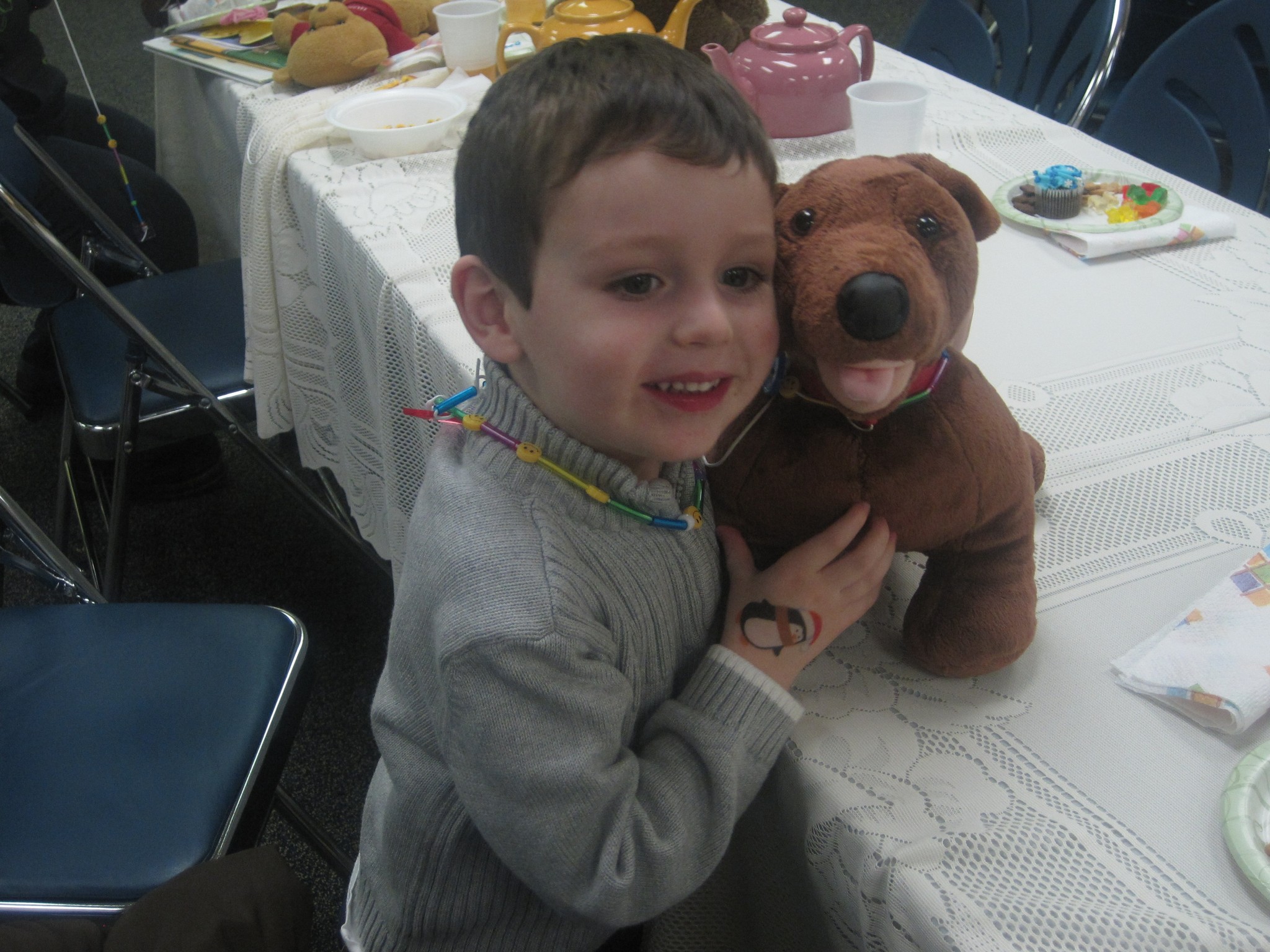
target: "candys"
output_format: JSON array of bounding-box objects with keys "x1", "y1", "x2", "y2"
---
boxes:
[{"x1": 1104, "y1": 181, "x2": 1168, "y2": 224}]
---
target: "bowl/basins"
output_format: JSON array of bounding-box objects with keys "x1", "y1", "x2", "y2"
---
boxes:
[{"x1": 331, "y1": 87, "x2": 466, "y2": 160}]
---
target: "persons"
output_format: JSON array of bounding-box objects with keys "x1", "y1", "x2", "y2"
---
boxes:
[
  {"x1": 338, "y1": 29, "x2": 895, "y2": 952},
  {"x1": 0, "y1": 0, "x2": 230, "y2": 503}
]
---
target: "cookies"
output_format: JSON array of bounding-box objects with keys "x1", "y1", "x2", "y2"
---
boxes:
[{"x1": 1012, "y1": 184, "x2": 1036, "y2": 216}]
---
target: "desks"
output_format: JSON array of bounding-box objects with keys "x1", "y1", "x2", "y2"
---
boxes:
[{"x1": 147, "y1": 0, "x2": 1269, "y2": 951}]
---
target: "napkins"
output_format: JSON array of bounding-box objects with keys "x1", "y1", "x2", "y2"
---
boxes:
[
  {"x1": 1049, "y1": 201, "x2": 1238, "y2": 263},
  {"x1": 1109, "y1": 549, "x2": 1268, "y2": 737}
]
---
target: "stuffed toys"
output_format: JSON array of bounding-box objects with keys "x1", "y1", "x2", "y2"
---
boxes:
[
  {"x1": 267, "y1": 0, "x2": 449, "y2": 87},
  {"x1": 703, "y1": 153, "x2": 1051, "y2": 674}
]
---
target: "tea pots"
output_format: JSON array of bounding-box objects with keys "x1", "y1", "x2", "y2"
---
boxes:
[
  {"x1": 701, "y1": 6, "x2": 876, "y2": 139},
  {"x1": 487, "y1": 0, "x2": 700, "y2": 75}
]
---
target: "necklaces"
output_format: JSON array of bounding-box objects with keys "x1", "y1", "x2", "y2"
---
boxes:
[{"x1": 402, "y1": 384, "x2": 705, "y2": 531}]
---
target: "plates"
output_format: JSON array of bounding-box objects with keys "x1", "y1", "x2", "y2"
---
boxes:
[
  {"x1": 1219, "y1": 741, "x2": 1269, "y2": 901},
  {"x1": 994, "y1": 167, "x2": 1186, "y2": 235}
]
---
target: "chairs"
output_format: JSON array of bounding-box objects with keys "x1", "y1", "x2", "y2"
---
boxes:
[
  {"x1": 1, "y1": 485, "x2": 357, "y2": 920},
  {"x1": 902, "y1": 0, "x2": 1133, "y2": 132},
  {"x1": 1088, "y1": 0, "x2": 1269, "y2": 218},
  {"x1": 0, "y1": 101, "x2": 399, "y2": 603}
]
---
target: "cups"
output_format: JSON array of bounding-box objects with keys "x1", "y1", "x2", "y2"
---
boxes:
[
  {"x1": 435, "y1": 1, "x2": 499, "y2": 71},
  {"x1": 845, "y1": 78, "x2": 929, "y2": 156}
]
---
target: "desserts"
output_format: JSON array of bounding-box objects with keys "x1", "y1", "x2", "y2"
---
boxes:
[{"x1": 1033, "y1": 164, "x2": 1084, "y2": 219}]
